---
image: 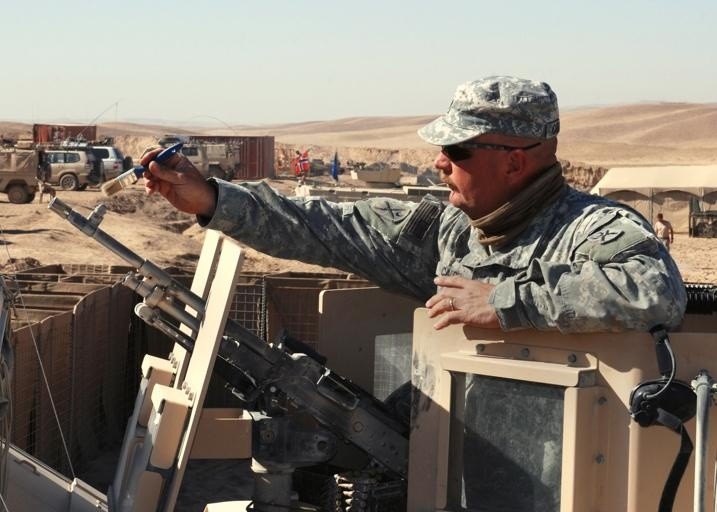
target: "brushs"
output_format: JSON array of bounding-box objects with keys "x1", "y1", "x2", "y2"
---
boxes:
[{"x1": 100, "y1": 141, "x2": 185, "y2": 197}]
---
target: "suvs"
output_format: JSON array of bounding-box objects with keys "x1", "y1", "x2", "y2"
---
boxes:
[
  {"x1": 176, "y1": 142, "x2": 241, "y2": 182},
  {"x1": 0, "y1": 146, "x2": 133, "y2": 204}
]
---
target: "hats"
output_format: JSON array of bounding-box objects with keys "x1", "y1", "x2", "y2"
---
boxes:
[{"x1": 416, "y1": 73, "x2": 560, "y2": 145}]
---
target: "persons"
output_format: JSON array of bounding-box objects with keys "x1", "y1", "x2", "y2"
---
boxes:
[
  {"x1": 653, "y1": 213, "x2": 674, "y2": 253},
  {"x1": 295, "y1": 148, "x2": 306, "y2": 187},
  {"x1": 138, "y1": 75, "x2": 688, "y2": 512}
]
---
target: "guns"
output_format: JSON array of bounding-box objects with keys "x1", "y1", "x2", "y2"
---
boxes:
[{"x1": 46, "y1": 197, "x2": 409, "y2": 510}]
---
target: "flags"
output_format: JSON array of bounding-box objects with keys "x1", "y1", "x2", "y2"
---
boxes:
[
  {"x1": 330, "y1": 149, "x2": 338, "y2": 185},
  {"x1": 293, "y1": 150, "x2": 311, "y2": 178}
]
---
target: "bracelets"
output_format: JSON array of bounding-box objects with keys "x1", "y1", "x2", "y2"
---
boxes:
[{"x1": 671, "y1": 238, "x2": 673, "y2": 240}]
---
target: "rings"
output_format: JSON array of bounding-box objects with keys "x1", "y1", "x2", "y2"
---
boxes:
[{"x1": 449, "y1": 296, "x2": 454, "y2": 311}]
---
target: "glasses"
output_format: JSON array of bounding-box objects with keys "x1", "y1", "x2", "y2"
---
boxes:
[{"x1": 442, "y1": 141, "x2": 542, "y2": 161}]
